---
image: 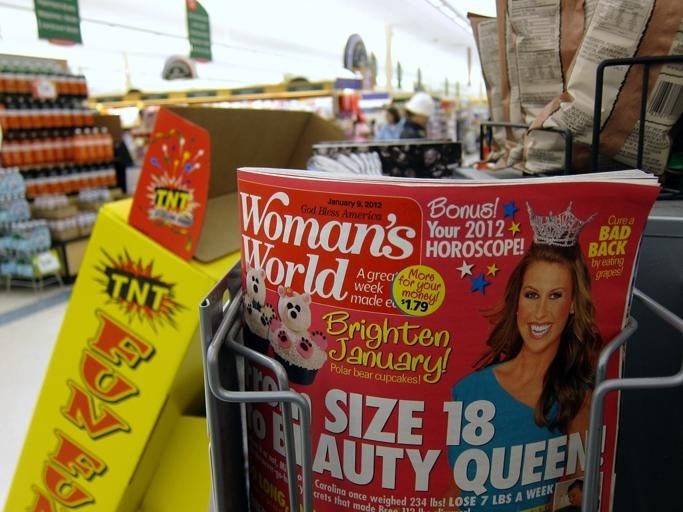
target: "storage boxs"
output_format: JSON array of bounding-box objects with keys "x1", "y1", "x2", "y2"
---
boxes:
[
  {"x1": 140, "y1": 415, "x2": 213, "y2": 512},
  {"x1": 51, "y1": 241, "x2": 89, "y2": 277},
  {"x1": 0, "y1": 198, "x2": 241, "y2": 511},
  {"x1": 18, "y1": 251, "x2": 61, "y2": 278}
]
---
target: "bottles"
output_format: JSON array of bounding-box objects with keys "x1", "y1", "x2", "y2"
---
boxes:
[
  {"x1": 101, "y1": 126, "x2": 114, "y2": 165},
  {"x1": 84, "y1": 127, "x2": 95, "y2": 165},
  {"x1": 74, "y1": 128, "x2": 88, "y2": 165},
  {"x1": 36, "y1": 191, "x2": 119, "y2": 242},
  {"x1": 0, "y1": 96, "x2": 94, "y2": 136},
  {"x1": 92, "y1": 127, "x2": 102, "y2": 165},
  {"x1": 23, "y1": 165, "x2": 117, "y2": 199},
  {"x1": 0, "y1": 58, "x2": 88, "y2": 102},
  {"x1": 0, "y1": 131, "x2": 74, "y2": 168},
  {"x1": 0, "y1": 167, "x2": 60, "y2": 281}
]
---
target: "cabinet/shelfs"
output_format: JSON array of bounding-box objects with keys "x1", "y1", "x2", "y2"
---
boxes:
[{"x1": 0, "y1": 54, "x2": 120, "y2": 239}]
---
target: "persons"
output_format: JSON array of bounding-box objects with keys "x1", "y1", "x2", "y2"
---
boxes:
[
  {"x1": 447, "y1": 228, "x2": 603, "y2": 512},
  {"x1": 464, "y1": 120, "x2": 477, "y2": 153},
  {"x1": 374, "y1": 107, "x2": 407, "y2": 140},
  {"x1": 555, "y1": 480, "x2": 600, "y2": 511},
  {"x1": 355, "y1": 112, "x2": 373, "y2": 141},
  {"x1": 399, "y1": 93, "x2": 434, "y2": 139},
  {"x1": 472, "y1": 113, "x2": 482, "y2": 134}
]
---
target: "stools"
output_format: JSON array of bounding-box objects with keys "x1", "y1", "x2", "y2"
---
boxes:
[{"x1": 6, "y1": 273, "x2": 63, "y2": 292}]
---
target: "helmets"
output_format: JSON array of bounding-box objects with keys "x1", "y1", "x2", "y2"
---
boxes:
[{"x1": 404, "y1": 93, "x2": 434, "y2": 116}]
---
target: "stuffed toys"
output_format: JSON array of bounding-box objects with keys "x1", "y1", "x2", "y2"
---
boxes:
[
  {"x1": 243, "y1": 262, "x2": 275, "y2": 326},
  {"x1": 269, "y1": 285, "x2": 313, "y2": 358}
]
